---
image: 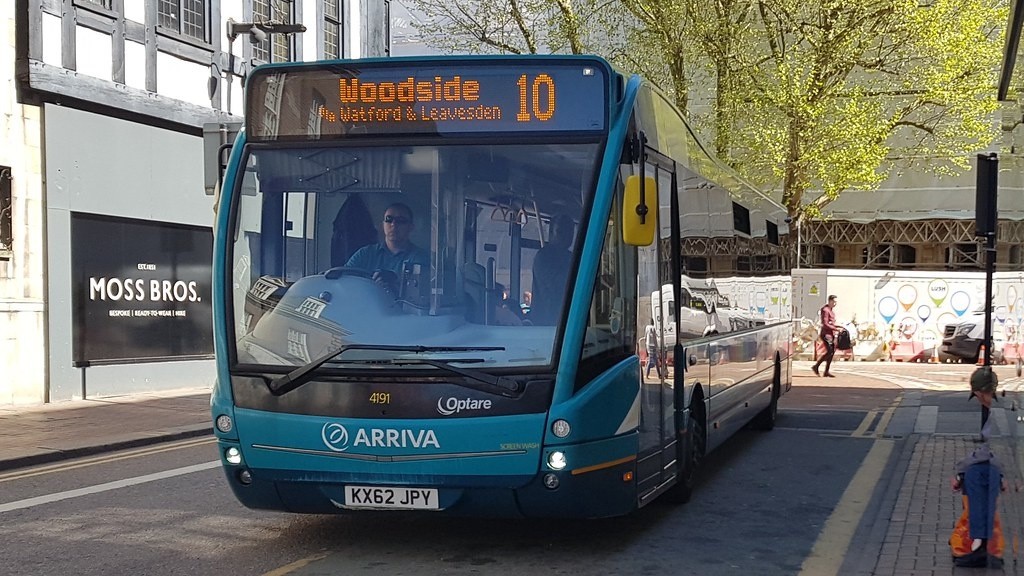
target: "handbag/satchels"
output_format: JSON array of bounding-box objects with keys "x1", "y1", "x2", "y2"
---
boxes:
[{"x1": 837, "y1": 331, "x2": 852, "y2": 349}]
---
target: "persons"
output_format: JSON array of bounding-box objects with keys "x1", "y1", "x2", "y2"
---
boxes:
[
  {"x1": 811, "y1": 294, "x2": 844, "y2": 377},
  {"x1": 332, "y1": 201, "x2": 433, "y2": 309},
  {"x1": 527, "y1": 215, "x2": 587, "y2": 326},
  {"x1": 644, "y1": 318, "x2": 664, "y2": 382}
]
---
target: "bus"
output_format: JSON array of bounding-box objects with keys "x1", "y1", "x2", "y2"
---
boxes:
[{"x1": 208, "y1": 49, "x2": 793, "y2": 533}]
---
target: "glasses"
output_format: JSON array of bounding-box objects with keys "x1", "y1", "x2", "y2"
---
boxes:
[{"x1": 384, "y1": 215, "x2": 411, "y2": 223}]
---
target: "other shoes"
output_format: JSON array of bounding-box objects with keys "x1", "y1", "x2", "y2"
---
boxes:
[
  {"x1": 813, "y1": 366, "x2": 821, "y2": 377},
  {"x1": 824, "y1": 372, "x2": 835, "y2": 377}
]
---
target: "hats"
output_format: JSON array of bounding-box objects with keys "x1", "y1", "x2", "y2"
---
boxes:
[{"x1": 968, "y1": 367, "x2": 997, "y2": 401}]
